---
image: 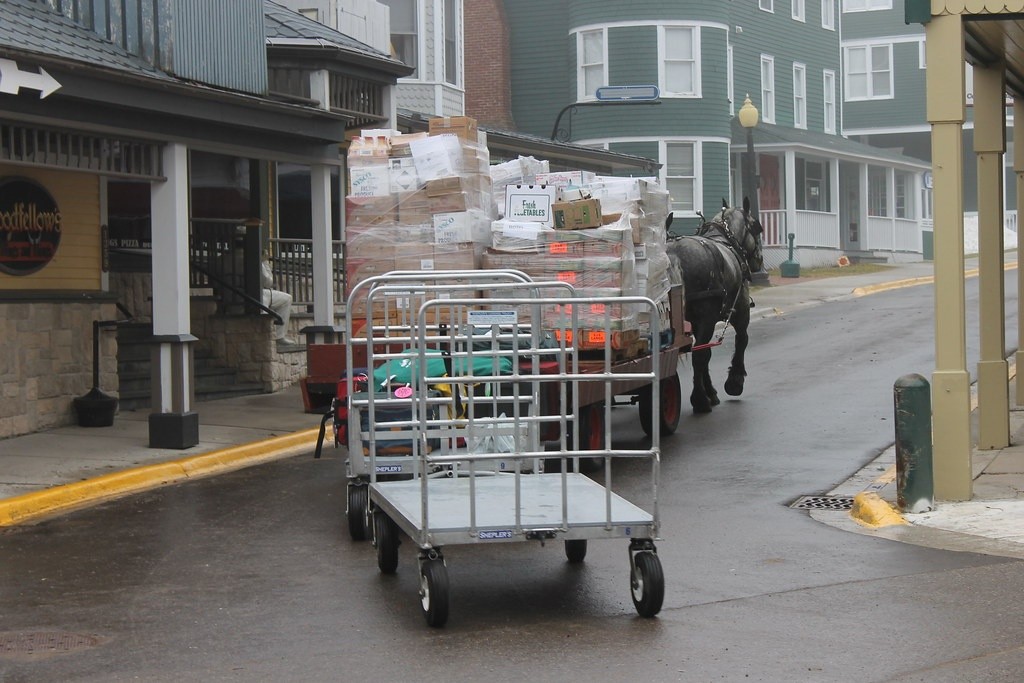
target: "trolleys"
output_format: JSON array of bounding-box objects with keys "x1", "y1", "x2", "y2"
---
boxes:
[{"x1": 346, "y1": 269, "x2": 667, "y2": 628}]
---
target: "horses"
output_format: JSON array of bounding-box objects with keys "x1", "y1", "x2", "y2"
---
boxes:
[{"x1": 664, "y1": 197, "x2": 764, "y2": 414}]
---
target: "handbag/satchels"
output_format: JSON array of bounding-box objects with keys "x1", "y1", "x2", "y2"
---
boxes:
[{"x1": 334, "y1": 365, "x2": 372, "y2": 451}]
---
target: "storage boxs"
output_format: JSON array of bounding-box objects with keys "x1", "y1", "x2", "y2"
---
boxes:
[
  {"x1": 355, "y1": 393, "x2": 440, "y2": 456},
  {"x1": 345, "y1": 114, "x2": 673, "y2": 361}
]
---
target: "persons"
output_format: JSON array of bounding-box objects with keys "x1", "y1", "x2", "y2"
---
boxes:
[{"x1": 212, "y1": 226, "x2": 297, "y2": 347}]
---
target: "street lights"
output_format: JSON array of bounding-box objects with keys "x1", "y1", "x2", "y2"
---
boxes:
[{"x1": 738, "y1": 93, "x2": 770, "y2": 286}]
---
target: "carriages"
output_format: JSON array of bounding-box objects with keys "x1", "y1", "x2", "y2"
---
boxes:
[{"x1": 300, "y1": 196, "x2": 763, "y2": 475}]
